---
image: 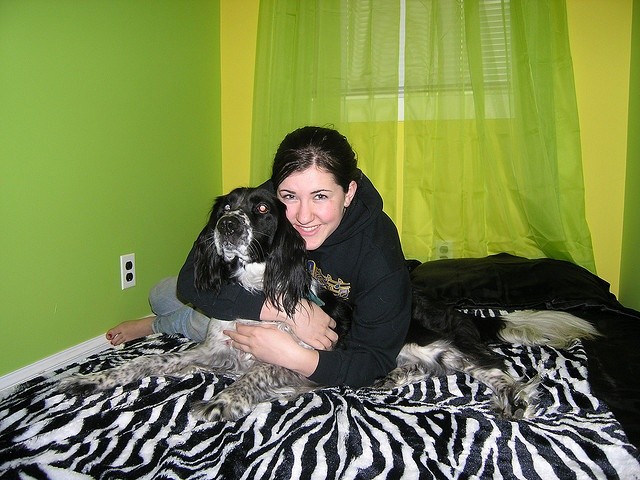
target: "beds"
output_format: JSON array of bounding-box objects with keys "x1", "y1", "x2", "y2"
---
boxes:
[{"x1": 1, "y1": 252, "x2": 640, "y2": 480}]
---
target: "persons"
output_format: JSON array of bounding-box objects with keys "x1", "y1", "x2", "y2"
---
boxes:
[{"x1": 106, "y1": 126, "x2": 413, "y2": 390}]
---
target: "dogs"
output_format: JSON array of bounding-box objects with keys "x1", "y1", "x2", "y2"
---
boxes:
[{"x1": 56, "y1": 185, "x2": 602, "y2": 425}]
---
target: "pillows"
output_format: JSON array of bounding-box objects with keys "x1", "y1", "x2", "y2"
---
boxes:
[{"x1": 410, "y1": 252, "x2": 623, "y2": 315}]
